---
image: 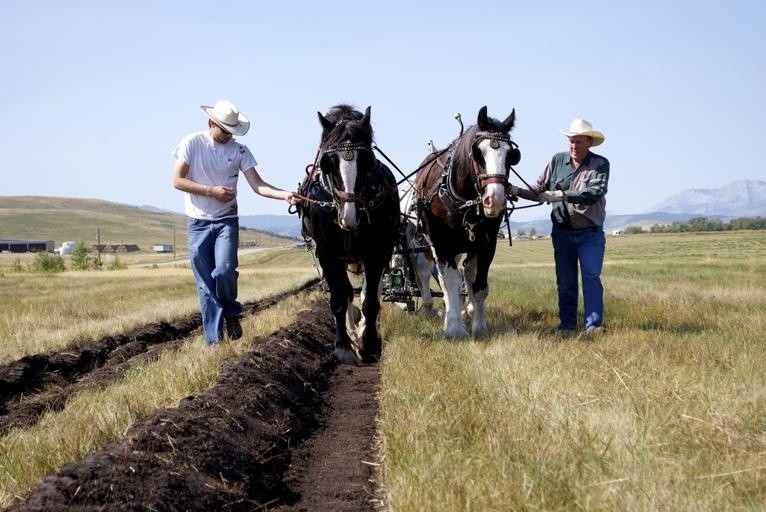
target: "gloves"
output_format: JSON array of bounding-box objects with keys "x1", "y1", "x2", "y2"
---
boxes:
[
  {"x1": 510, "y1": 185, "x2": 518, "y2": 196},
  {"x1": 536, "y1": 190, "x2": 563, "y2": 204}
]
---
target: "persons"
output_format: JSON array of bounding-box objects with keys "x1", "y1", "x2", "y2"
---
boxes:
[
  {"x1": 508, "y1": 118, "x2": 609, "y2": 333},
  {"x1": 171, "y1": 99, "x2": 301, "y2": 345}
]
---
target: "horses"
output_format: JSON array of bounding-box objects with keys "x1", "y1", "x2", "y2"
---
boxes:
[
  {"x1": 303, "y1": 106, "x2": 400, "y2": 364},
  {"x1": 405, "y1": 105, "x2": 516, "y2": 340}
]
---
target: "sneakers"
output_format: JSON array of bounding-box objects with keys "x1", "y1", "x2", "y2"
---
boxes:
[
  {"x1": 224, "y1": 316, "x2": 242, "y2": 341},
  {"x1": 590, "y1": 327, "x2": 606, "y2": 335}
]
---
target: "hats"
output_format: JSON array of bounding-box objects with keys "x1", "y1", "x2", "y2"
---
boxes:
[
  {"x1": 200, "y1": 100, "x2": 249, "y2": 136},
  {"x1": 562, "y1": 119, "x2": 604, "y2": 146}
]
